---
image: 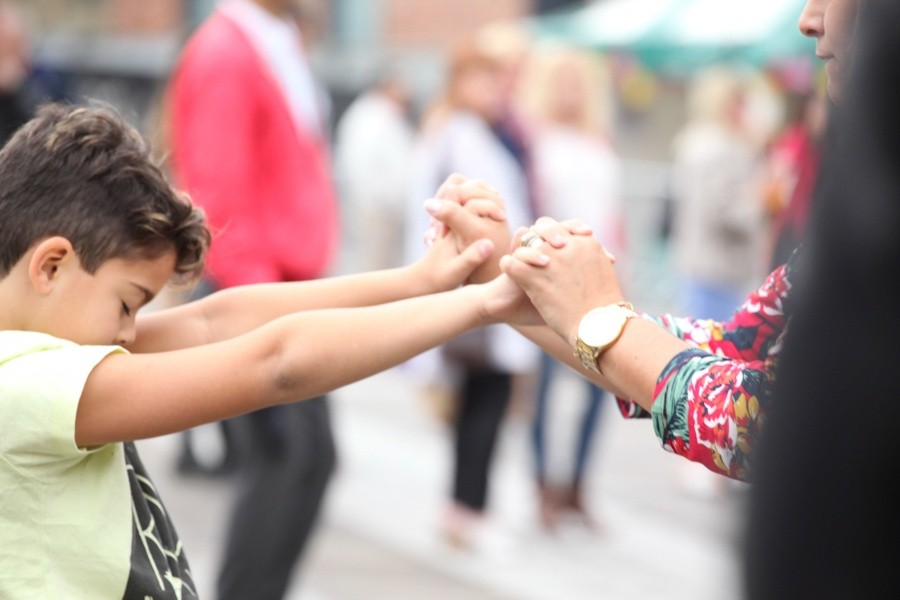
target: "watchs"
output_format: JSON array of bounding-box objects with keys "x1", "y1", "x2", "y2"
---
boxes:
[{"x1": 574, "y1": 302, "x2": 637, "y2": 374}]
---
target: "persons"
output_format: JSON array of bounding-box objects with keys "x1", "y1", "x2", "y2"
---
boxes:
[{"x1": 1, "y1": 0, "x2": 900, "y2": 600}]
